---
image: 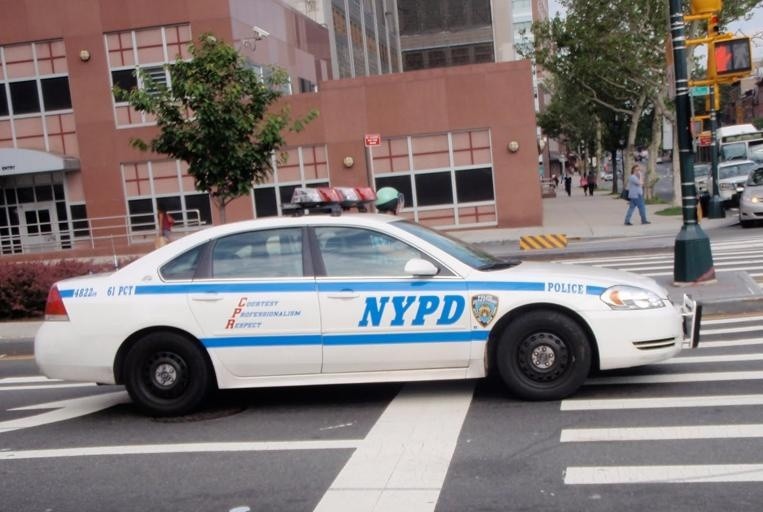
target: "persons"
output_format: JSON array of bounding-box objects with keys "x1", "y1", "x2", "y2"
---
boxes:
[
  {"x1": 553, "y1": 161, "x2": 608, "y2": 196},
  {"x1": 623, "y1": 165, "x2": 652, "y2": 226},
  {"x1": 155, "y1": 204, "x2": 172, "y2": 248},
  {"x1": 369, "y1": 186, "x2": 425, "y2": 266}
]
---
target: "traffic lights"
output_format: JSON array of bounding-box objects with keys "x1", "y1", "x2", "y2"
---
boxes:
[{"x1": 708, "y1": 35, "x2": 755, "y2": 83}]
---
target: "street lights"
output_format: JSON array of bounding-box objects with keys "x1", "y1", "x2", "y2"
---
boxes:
[{"x1": 558, "y1": 155, "x2": 567, "y2": 174}]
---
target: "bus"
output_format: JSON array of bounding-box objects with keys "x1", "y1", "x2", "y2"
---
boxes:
[{"x1": 694, "y1": 123, "x2": 762, "y2": 166}]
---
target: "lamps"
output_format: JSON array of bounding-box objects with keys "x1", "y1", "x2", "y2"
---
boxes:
[
  {"x1": 344, "y1": 156, "x2": 353, "y2": 167},
  {"x1": 78, "y1": 50, "x2": 91, "y2": 61},
  {"x1": 507, "y1": 141, "x2": 519, "y2": 154}
]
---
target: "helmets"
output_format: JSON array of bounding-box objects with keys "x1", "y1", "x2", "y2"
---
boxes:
[{"x1": 373, "y1": 186, "x2": 405, "y2": 215}]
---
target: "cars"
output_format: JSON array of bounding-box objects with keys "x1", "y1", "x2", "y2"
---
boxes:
[
  {"x1": 693, "y1": 163, "x2": 710, "y2": 195},
  {"x1": 26, "y1": 185, "x2": 703, "y2": 414},
  {"x1": 736, "y1": 164, "x2": 763, "y2": 229},
  {"x1": 707, "y1": 159, "x2": 759, "y2": 205}
]
